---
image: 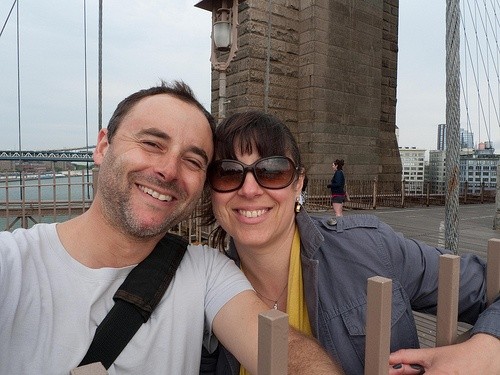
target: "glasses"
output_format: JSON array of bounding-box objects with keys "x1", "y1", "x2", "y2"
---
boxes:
[{"x1": 205, "y1": 155, "x2": 299, "y2": 193}]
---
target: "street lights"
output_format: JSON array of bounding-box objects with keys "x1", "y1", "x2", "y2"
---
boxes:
[{"x1": 192, "y1": 0, "x2": 251, "y2": 126}]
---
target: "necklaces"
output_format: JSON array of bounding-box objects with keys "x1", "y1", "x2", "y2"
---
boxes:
[{"x1": 256, "y1": 285, "x2": 287, "y2": 310}]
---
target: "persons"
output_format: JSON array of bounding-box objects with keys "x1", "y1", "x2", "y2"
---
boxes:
[
  {"x1": 1, "y1": 81, "x2": 347, "y2": 375},
  {"x1": 188, "y1": 111, "x2": 499, "y2": 375},
  {"x1": 327, "y1": 159, "x2": 346, "y2": 217}
]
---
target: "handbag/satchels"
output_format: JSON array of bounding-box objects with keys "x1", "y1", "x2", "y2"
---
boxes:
[{"x1": 344, "y1": 192, "x2": 350, "y2": 202}]
